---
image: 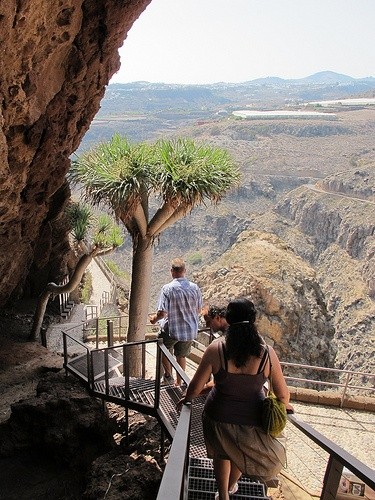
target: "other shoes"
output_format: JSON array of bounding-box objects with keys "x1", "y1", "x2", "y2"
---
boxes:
[
  {"x1": 228, "y1": 482, "x2": 239, "y2": 496},
  {"x1": 160, "y1": 375, "x2": 173, "y2": 385}
]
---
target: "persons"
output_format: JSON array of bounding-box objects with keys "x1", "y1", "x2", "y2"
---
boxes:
[
  {"x1": 173, "y1": 296, "x2": 297, "y2": 500},
  {"x1": 147, "y1": 258, "x2": 203, "y2": 387},
  {"x1": 198, "y1": 302, "x2": 270, "y2": 396}
]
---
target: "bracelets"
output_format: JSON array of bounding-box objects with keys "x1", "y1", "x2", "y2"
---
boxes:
[{"x1": 181, "y1": 397, "x2": 192, "y2": 405}]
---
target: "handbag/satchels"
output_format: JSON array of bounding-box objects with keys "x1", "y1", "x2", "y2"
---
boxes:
[
  {"x1": 261, "y1": 386, "x2": 290, "y2": 438},
  {"x1": 214, "y1": 491, "x2": 219, "y2": 500}
]
